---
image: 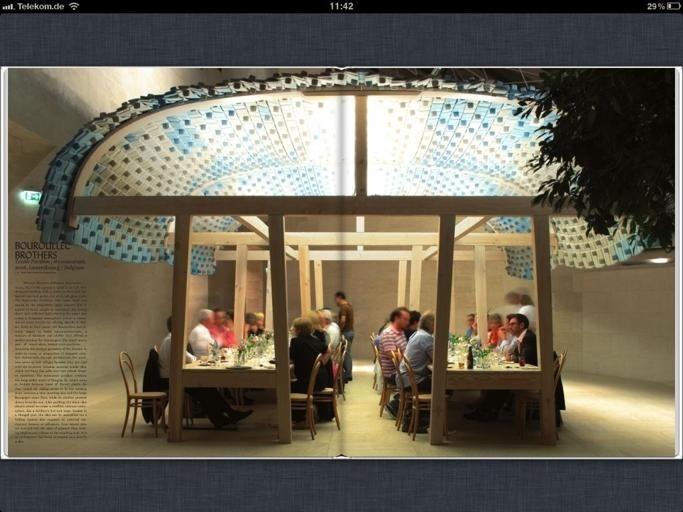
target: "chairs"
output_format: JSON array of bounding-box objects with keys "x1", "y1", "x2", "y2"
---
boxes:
[
  {"x1": 369, "y1": 331, "x2": 447, "y2": 441},
  {"x1": 152, "y1": 345, "x2": 193, "y2": 428},
  {"x1": 290, "y1": 334, "x2": 348, "y2": 440},
  {"x1": 119, "y1": 352, "x2": 169, "y2": 438},
  {"x1": 526, "y1": 347, "x2": 568, "y2": 440}
]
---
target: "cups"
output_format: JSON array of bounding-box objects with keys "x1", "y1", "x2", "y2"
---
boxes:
[{"x1": 194, "y1": 328, "x2": 272, "y2": 368}]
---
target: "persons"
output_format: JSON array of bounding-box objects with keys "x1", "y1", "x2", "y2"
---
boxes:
[
  {"x1": 372, "y1": 287, "x2": 565, "y2": 433},
  {"x1": 157, "y1": 291, "x2": 353, "y2": 430}
]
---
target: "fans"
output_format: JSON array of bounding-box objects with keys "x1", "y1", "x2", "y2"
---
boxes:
[{"x1": 182, "y1": 331, "x2": 293, "y2": 440}]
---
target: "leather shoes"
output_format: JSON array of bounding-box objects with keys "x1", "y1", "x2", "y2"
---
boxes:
[{"x1": 215, "y1": 407, "x2": 253, "y2": 427}]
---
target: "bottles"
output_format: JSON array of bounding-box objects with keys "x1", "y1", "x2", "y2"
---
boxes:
[{"x1": 445, "y1": 333, "x2": 526, "y2": 369}]
